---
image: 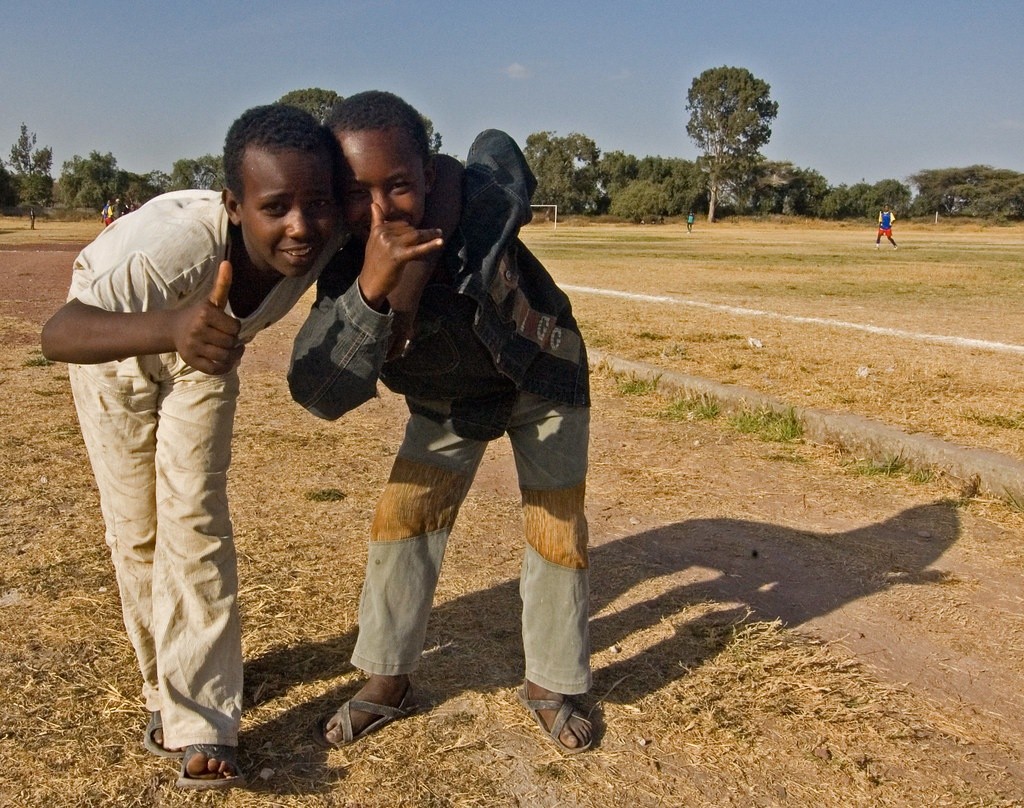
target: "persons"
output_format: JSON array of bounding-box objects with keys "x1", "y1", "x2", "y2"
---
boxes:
[
  {"x1": 30, "y1": 209, "x2": 35, "y2": 230},
  {"x1": 876, "y1": 204, "x2": 898, "y2": 251},
  {"x1": 40, "y1": 99, "x2": 339, "y2": 790},
  {"x1": 687, "y1": 211, "x2": 694, "y2": 234},
  {"x1": 100, "y1": 197, "x2": 135, "y2": 226},
  {"x1": 287, "y1": 90, "x2": 596, "y2": 753}
]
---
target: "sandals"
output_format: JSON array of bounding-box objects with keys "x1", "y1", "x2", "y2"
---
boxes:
[
  {"x1": 143, "y1": 710, "x2": 183, "y2": 758},
  {"x1": 517, "y1": 677, "x2": 591, "y2": 755},
  {"x1": 313, "y1": 680, "x2": 420, "y2": 747},
  {"x1": 176, "y1": 744, "x2": 244, "y2": 790}
]
